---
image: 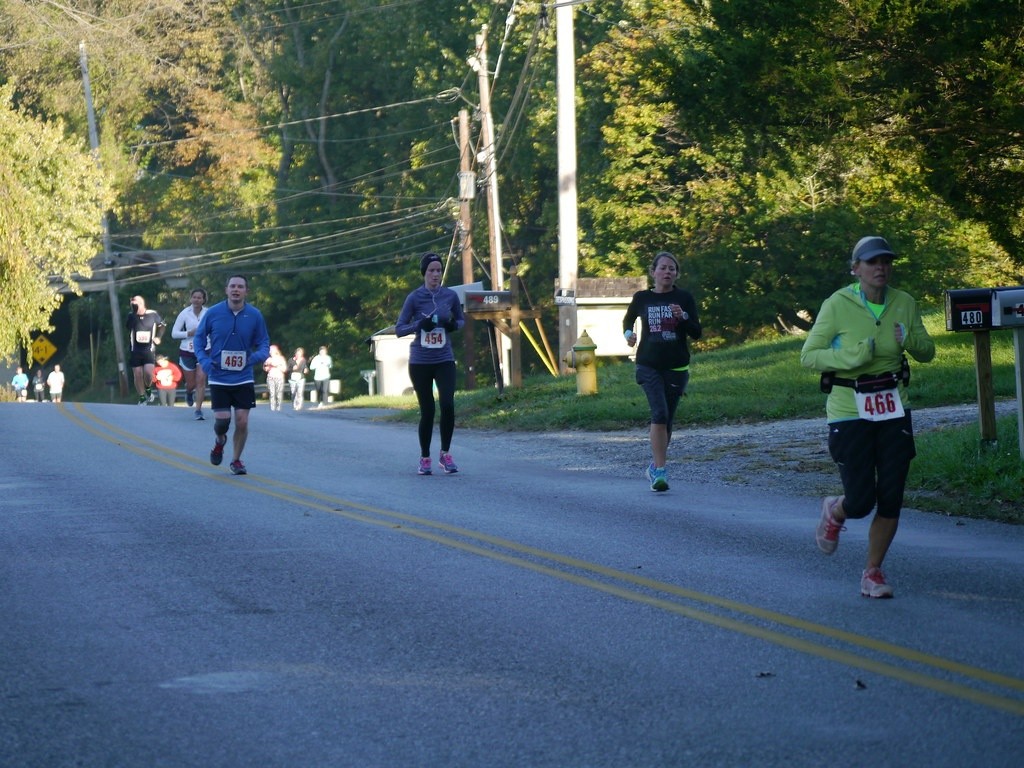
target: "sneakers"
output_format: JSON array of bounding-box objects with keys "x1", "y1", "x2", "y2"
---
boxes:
[
  {"x1": 417, "y1": 457, "x2": 434, "y2": 475},
  {"x1": 229, "y1": 460, "x2": 247, "y2": 474},
  {"x1": 210, "y1": 435, "x2": 227, "y2": 465},
  {"x1": 645, "y1": 462, "x2": 670, "y2": 492},
  {"x1": 194, "y1": 410, "x2": 205, "y2": 419},
  {"x1": 438, "y1": 449, "x2": 460, "y2": 473},
  {"x1": 186, "y1": 390, "x2": 194, "y2": 406},
  {"x1": 815, "y1": 495, "x2": 848, "y2": 554},
  {"x1": 860, "y1": 566, "x2": 894, "y2": 598}
]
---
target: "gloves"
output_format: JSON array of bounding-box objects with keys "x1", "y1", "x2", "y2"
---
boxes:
[
  {"x1": 443, "y1": 317, "x2": 456, "y2": 332},
  {"x1": 421, "y1": 314, "x2": 436, "y2": 332}
]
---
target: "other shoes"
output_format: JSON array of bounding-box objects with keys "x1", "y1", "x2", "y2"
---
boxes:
[
  {"x1": 138, "y1": 401, "x2": 148, "y2": 407},
  {"x1": 146, "y1": 393, "x2": 154, "y2": 402}
]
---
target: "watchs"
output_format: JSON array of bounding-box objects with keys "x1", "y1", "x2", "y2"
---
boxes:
[{"x1": 678, "y1": 311, "x2": 689, "y2": 323}]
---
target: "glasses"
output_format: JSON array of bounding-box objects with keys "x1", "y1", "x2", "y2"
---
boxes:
[{"x1": 130, "y1": 298, "x2": 136, "y2": 301}]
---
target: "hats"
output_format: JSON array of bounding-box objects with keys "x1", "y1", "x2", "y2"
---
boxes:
[{"x1": 852, "y1": 238, "x2": 899, "y2": 262}]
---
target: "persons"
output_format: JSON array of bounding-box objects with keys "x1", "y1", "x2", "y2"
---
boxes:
[
  {"x1": 126, "y1": 295, "x2": 166, "y2": 406},
  {"x1": 310, "y1": 346, "x2": 334, "y2": 408},
  {"x1": 394, "y1": 252, "x2": 466, "y2": 475},
  {"x1": 32, "y1": 369, "x2": 46, "y2": 402},
  {"x1": 11, "y1": 367, "x2": 29, "y2": 402},
  {"x1": 194, "y1": 275, "x2": 270, "y2": 474},
  {"x1": 262, "y1": 345, "x2": 287, "y2": 412},
  {"x1": 802, "y1": 237, "x2": 935, "y2": 597},
  {"x1": 286, "y1": 347, "x2": 310, "y2": 411},
  {"x1": 171, "y1": 289, "x2": 211, "y2": 420},
  {"x1": 623, "y1": 250, "x2": 701, "y2": 492},
  {"x1": 47, "y1": 364, "x2": 64, "y2": 403},
  {"x1": 152, "y1": 354, "x2": 182, "y2": 406}
]
